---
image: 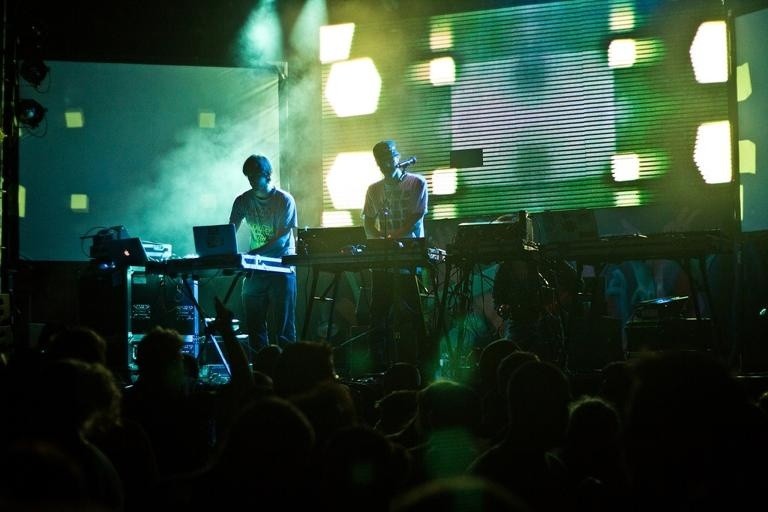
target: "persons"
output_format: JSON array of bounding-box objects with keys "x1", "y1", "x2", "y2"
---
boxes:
[
  {"x1": 225, "y1": 154, "x2": 299, "y2": 370},
  {"x1": 360, "y1": 137, "x2": 439, "y2": 386}
]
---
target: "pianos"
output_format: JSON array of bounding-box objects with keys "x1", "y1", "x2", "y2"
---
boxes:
[
  {"x1": 537, "y1": 233, "x2": 730, "y2": 260},
  {"x1": 296, "y1": 238, "x2": 447, "y2": 269},
  {"x1": 146, "y1": 254, "x2": 291, "y2": 280}
]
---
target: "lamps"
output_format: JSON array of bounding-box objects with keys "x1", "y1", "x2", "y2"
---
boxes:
[
  {"x1": 12, "y1": 99, "x2": 48, "y2": 127},
  {"x1": 18, "y1": 52, "x2": 50, "y2": 87}
]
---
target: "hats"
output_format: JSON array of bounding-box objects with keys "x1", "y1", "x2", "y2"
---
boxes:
[{"x1": 372, "y1": 139, "x2": 400, "y2": 161}]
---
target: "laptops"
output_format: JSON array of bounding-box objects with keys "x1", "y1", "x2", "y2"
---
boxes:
[
  {"x1": 105, "y1": 238, "x2": 149, "y2": 266},
  {"x1": 193, "y1": 224, "x2": 237, "y2": 256}
]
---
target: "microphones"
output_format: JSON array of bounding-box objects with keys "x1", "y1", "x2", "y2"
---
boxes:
[{"x1": 396, "y1": 157, "x2": 417, "y2": 168}]
